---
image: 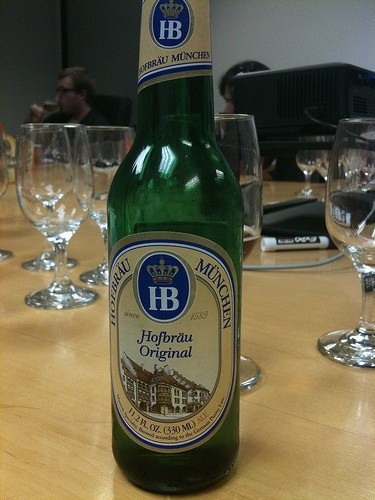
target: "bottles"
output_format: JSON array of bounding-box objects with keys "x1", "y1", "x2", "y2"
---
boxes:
[{"x1": 105, "y1": 1, "x2": 244, "y2": 497}]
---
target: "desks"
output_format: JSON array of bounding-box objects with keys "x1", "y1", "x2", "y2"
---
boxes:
[{"x1": 0, "y1": 156, "x2": 375, "y2": 500}]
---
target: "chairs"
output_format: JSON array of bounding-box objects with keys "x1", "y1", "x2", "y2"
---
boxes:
[{"x1": 92, "y1": 94, "x2": 132, "y2": 142}]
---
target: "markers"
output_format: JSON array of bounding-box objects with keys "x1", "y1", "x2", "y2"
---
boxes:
[{"x1": 261, "y1": 235, "x2": 339, "y2": 252}]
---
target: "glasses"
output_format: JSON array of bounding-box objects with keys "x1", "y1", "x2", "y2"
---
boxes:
[
  {"x1": 55, "y1": 88, "x2": 76, "y2": 96},
  {"x1": 221, "y1": 98, "x2": 233, "y2": 104}
]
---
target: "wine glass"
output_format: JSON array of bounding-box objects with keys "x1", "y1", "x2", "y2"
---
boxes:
[
  {"x1": 315, "y1": 116, "x2": 375, "y2": 369},
  {"x1": 293, "y1": 134, "x2": 332, "y2": 199},
  {"x1": 212, "y1": 113, "x2": 265, "y2": 398},
  {"x1": 30, "y1": 99, "x2": 62, "y2": 127},
  {"x1": 13, "y1": 123, "x2": 100, "y2": 311},
  {"x1": 0, "y1": 129, "x2": 15, "y2": 261},
  {"x1": 71, "y1": 124, "x2": 134, "y2": 286}
]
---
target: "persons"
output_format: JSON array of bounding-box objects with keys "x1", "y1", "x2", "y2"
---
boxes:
[
  {"x1": 219, "y1": 60, "x2": 271, "y2": 114},
  {"x1": 24, "y1": 67, "x2": 115, "y2": 159}
]
